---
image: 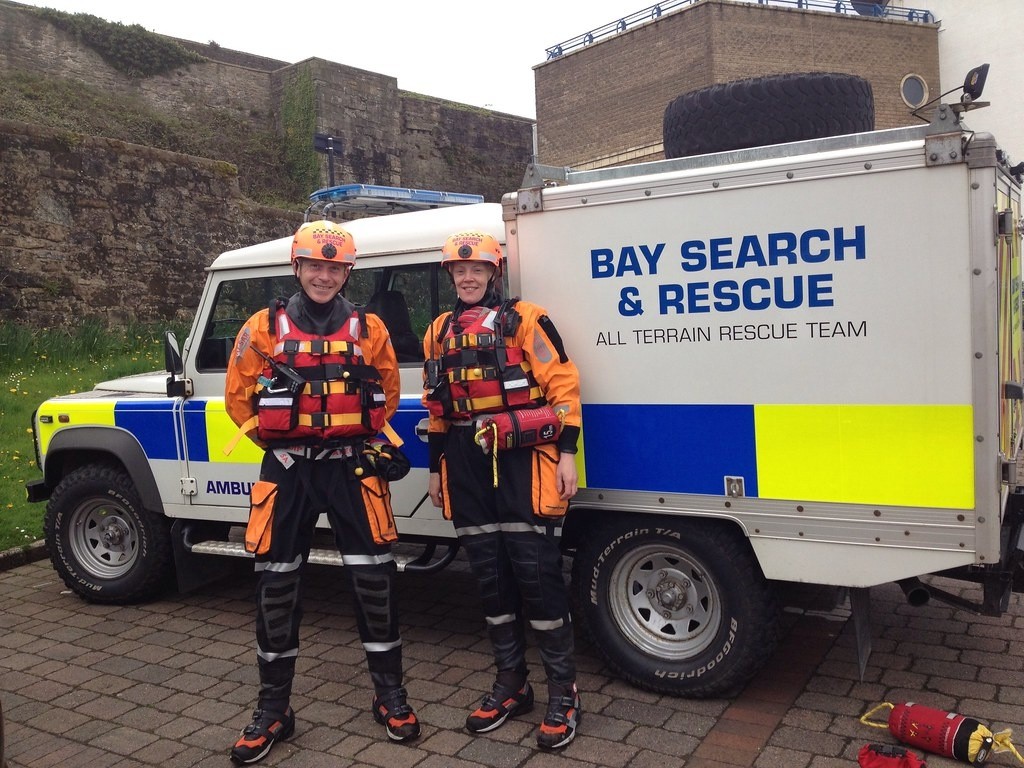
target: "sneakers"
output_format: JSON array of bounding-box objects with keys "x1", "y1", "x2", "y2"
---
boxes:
[
  {"x1": 466, "y1": 681, "x2": 534, "y2": 733},
  {"x1": 536, "y1": 690, "x2": 581, "y2": 749},
  {"x1": 371, "y1": 686, "x2": 420, "y2": 741},
  {"x1": 229, "y1": 704, "x2": 295, "y2": 765}
]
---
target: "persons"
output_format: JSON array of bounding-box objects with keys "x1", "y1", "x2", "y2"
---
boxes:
[
  {"x1": 224, "y1": 220, "x2": 424, "y2": 767},
  {"x1": 422, "y1": 229, "x2": 584, "y2": 751}
]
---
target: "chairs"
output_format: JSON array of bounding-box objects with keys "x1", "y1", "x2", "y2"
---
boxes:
[{"x1": 365, "y1": 291, "x2": 421, "y2": 364}]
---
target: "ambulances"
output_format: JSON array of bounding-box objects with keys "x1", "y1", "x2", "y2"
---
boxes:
[{"x1": 23, "y1": 62, "x2": 1024, "y2": 702}]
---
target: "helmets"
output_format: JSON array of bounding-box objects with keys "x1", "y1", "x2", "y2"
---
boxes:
[
  {"x1": 441, "y1": 231, "x2": 504, "y2": 277},
  {"x1": 291, "y1": 220, "x2": 356, "y2": 265}
]
